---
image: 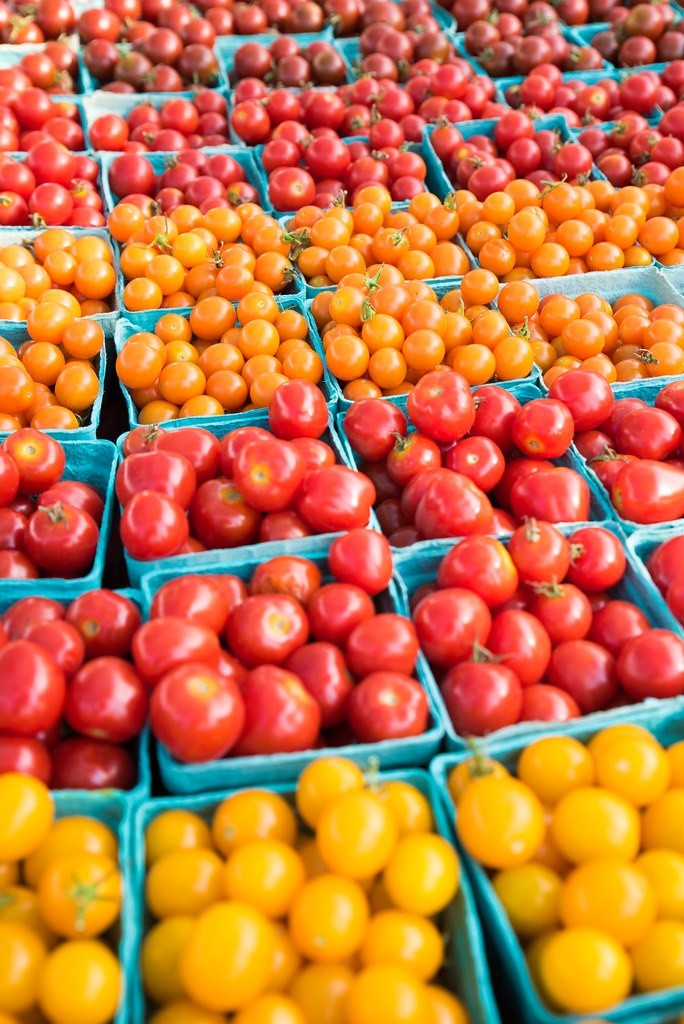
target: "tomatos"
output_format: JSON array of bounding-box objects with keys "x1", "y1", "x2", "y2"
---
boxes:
[{"x1": 0, "y1": 0, "x2": 684, "y2": 1024}]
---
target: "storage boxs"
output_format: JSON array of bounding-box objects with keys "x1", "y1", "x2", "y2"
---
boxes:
[{"x1": 0, "y1": 0, "x2": 684, "y2": 1024}]
